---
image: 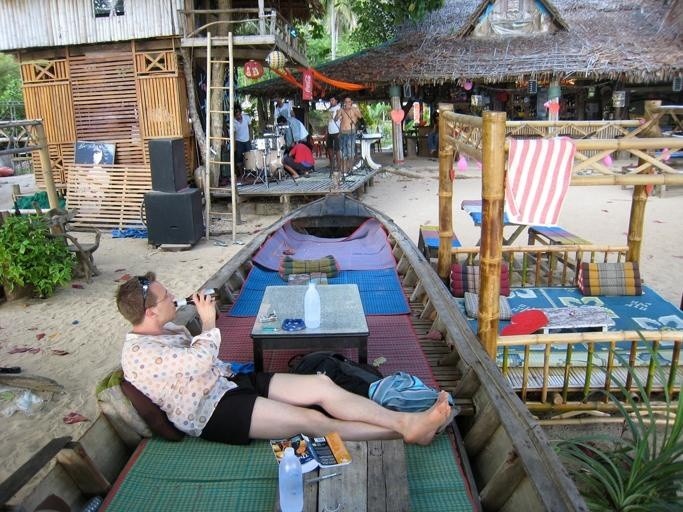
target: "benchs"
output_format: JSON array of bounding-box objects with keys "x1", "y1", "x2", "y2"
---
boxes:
[
  {"x1": 528, "y1": 226, "x2": 595, "y2": 277},
  {"x1": 418, "y1": 225, "x2": 460, "y2": 265},
  {"x1": 622, "y1": 166, "x2": 638, "y2": 190}
]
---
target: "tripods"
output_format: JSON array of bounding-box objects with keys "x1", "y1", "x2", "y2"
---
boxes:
[
  {"x1": 252, "y1": 127, "x2": 298, "y2": 189},
  {"x1": 324, "y1": 102, "x2": 375, "y2": 186}
]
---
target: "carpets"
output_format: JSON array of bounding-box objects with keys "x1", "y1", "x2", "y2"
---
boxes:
[
  {"x1": 100, "y1": 409, "x2": 480, "y2": 511},
  {"x1": 199, "y1": 314, "x2": 446, "y2": 407},
  {"x1": 255, "y1": 221, "x2": 399, "y2": 269},
  {"x1": 229, "y1": 263, "x2": 414, "y2": 316}
]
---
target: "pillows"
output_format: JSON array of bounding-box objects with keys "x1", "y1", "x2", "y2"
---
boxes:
[
  {"x1": 578, "y1": 261, "x2": 642, "y2": 295},
  {"x1": 450, "y1": 264, "x2": 510, "y2": 297},
  {"x1": 465, "y1": 292, "x2": 512, "y2": 321}
]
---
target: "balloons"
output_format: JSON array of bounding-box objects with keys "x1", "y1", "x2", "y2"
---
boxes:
[
  {"x1": 457, "y1": 158, "x2": 468, "y2": 172},
  {"x1": 463, "y1": 83, "x2": 472, "y2": 90},
  {"x1": 662, "y1": 148, "x2": 670, "y2": 160},
  {"x1": 603, "y1": 155, "x2": 612, "y2": 166}
]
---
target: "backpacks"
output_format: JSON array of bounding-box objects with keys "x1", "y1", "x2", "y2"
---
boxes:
[{"x1": 288, "y1": 350, "x2": 383, "y2": 398}]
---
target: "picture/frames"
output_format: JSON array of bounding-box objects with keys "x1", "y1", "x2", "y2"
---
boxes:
[{"x1": 74, "y1": 140, "x2": 116, "y2": 165}]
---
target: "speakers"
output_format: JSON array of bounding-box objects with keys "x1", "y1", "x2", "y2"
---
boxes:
[
  {"x1": 403, "y1": 83, "x2": 411, "y2": 99},
  {"x1": 148, "y1": 136, "x2": 188, "y2": 194},
  {"x1": 527, "y1": 79, "x2": 538, "y2": 95},
  {"x1": 146, "y1": 186, "x2": 203, "y2": 245}
]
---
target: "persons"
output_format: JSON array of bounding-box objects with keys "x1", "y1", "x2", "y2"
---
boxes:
[
  {"x1": 232, "y1": 107, "x2": 252, "y2": 164},
  {"x1": 283, "y1": 142, "x2": 314, "y2": 178},
  {"x1": 427, "y1": 111, "x2": 438, "y2": 154},
  {"x1": 333, "y1": 96, "x2": 361, "y2": 173},
  {"x1": 276, "y1": 116, "x2": 308, "y2": 144},
  {"x1": 325, "y1": 95, "x2": 342, "y2": 169},
  {"x1": 116, "y1": 271, "x2": 450, "y2": 446},
  {"x1": 272, "y1": 97, "x2": 294, "y2": 124}
]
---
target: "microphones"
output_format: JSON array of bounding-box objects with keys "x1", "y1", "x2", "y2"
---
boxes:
[{"x1": 318, "y1": 99, "x2": 326, "y2": 105}]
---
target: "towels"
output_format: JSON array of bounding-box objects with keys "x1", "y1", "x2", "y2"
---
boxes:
[{"x1": 506, "y1": 136, "x2": 576, "y2": 226}]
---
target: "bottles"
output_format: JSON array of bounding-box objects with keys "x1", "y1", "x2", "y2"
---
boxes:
[
  {"x1": 304, "y1": 283, "x2": 321, "y2": 328},
  {"x1": 173, "y1": 287, "x2": 221, "y2": 307},
  {"x1": 279, "y1": 447, "x2": 303, "y2": 512}
]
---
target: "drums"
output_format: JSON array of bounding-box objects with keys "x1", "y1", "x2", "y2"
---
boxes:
[
  {"x1": 266, "y1": 149, "x2": 285, "y2": 170},
  {"x1": 242, "y1": 149, "x2": 265, "y2": 171},
  {"x1": 251, "y1": 138, "x2": 273, "y2": 150},
  {"x1": 273, "y1": 135, "x2": 285, "y2": 150}
]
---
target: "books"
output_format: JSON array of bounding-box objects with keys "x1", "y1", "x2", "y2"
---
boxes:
[{"x1": 269, "y1": 432, "x2": 353, "y2": 473}]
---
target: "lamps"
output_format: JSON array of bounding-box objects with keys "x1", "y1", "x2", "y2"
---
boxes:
[{"x1": 612, "y1": 91, "x2": 625, "y2": 108}]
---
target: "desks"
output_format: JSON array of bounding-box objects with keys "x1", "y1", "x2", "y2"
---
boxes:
[
  {"x1": 250, "y1": 284, "x2": 371, "y2": 370},
  {"x1": 538, "y1": 306, "x2": 615, "y2": 335},
  {"x1": 312, "y1": 136, "x2": 326, "y2": 158},
  {"x1": 655, "y1": 152, "x2": 683, "y2": 198},
  {"x1": 461, "y1": 199, "x2": 531, "y2": 284},
  {"x1": 352, "y1": 133, "x2": 383, "y2": 170}
]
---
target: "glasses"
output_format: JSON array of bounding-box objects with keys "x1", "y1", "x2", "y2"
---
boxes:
[{"x1": 138, "y1": 273, "x2": 150, "y2": 311}]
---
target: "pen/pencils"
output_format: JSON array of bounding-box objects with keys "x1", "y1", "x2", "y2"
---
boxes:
[{"x1": 306, "y1": 473, "x2": 342, "y2": 483}]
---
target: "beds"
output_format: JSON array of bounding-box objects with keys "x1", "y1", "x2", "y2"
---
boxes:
[{"x1": 438, "y1": 98, "x2": 683, "y2": 426}]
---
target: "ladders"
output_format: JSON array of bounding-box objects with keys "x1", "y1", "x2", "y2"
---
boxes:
[{"x1": 205, "y1": 32, "x2": 237, "y2": 243}]
